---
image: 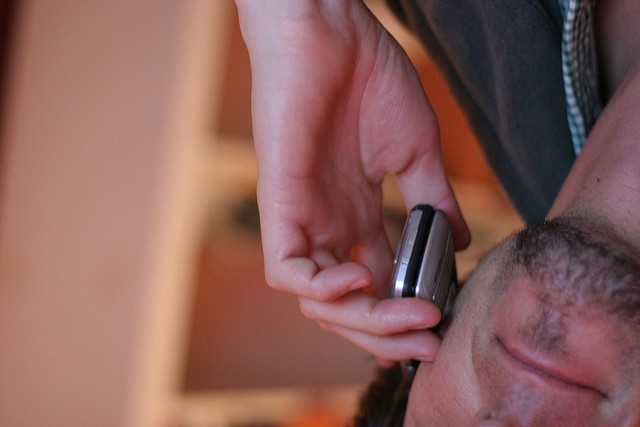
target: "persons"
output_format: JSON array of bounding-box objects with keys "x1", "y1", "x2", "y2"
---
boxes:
[{"x1": 234, "y1": 0, "x2": 638, "y2": 427}]
